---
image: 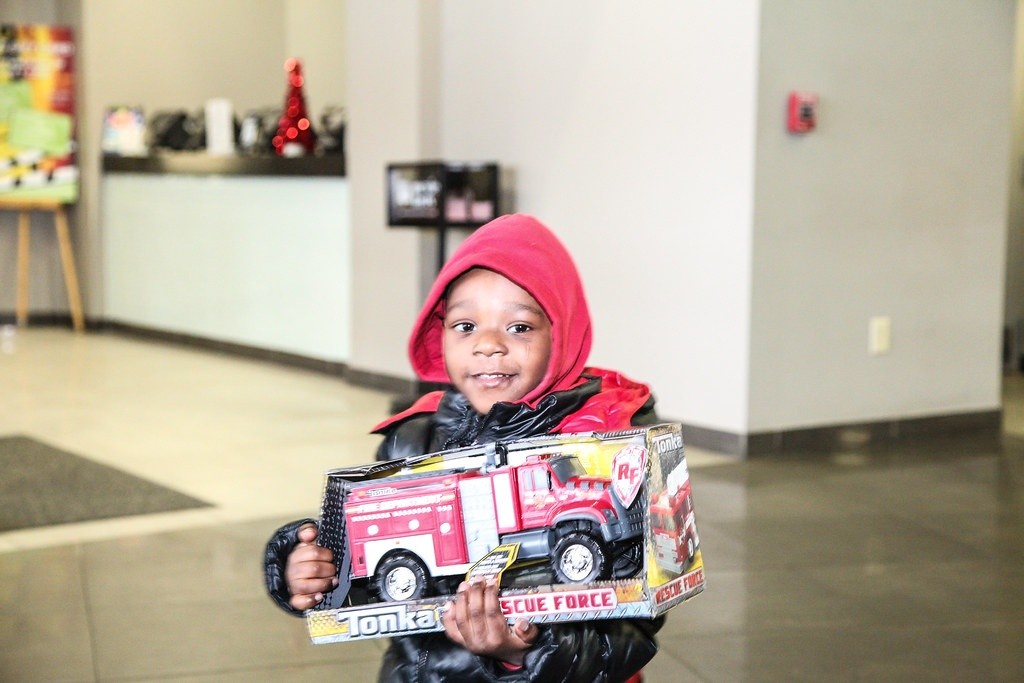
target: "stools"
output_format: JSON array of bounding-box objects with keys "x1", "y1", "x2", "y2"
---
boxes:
[{"x1": 0, "y1": 199, "x2": 87, "y2": 333}]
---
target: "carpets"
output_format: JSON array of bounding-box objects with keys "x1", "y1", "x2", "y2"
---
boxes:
[{"x1": 0, "y1": 431, "x2": 224, "y2": 534}]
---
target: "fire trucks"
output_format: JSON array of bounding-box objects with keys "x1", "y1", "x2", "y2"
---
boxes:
[{"x1": 349, "y1": 452, "x2": 644, "y2": 607}]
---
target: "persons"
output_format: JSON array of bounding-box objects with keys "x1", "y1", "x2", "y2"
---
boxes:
[{"x1": 262, "y1": 213, "x2": 666, "y2": 683}]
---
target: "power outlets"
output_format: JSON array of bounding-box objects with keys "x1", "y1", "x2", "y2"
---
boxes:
[{"x1": 869, "y1": 318, "x2": 890, "y2": 354}]
